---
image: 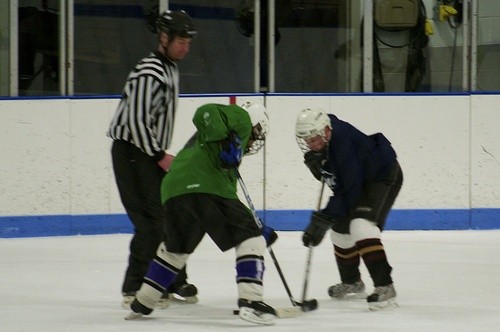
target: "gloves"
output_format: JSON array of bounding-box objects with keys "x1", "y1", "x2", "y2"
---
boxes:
[
  {"x1": 303, "y1": 150, "x2": 323, "y2": 181},
  {"x1": 220, "y1": 133, "x2": 242, "y2": 168},
  {"x1": 259, "y1": 224, "x2": 278, "y2": 247},
  {"x1": 302, "y1": 208, "x2": 335, "y2": 247}
]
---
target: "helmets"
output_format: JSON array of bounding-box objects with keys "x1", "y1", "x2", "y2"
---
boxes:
[
  {"x1": 241, "y1": 101, "x2": 271, "y2": 156},
  {"x1": 296, "y1": 109, "x2": 332, "y2": 155},
  {"x1": 154, "y1": 10, "x2": 199, "y2": 39}
]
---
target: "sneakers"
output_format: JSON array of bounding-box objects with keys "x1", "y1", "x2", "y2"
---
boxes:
[
  {"x1": 327, "y1": 280, "x2": 366, "y2": 300},
  {"x1": 366, "y1": 284, "x2": 399, "y2": 311},
  {"x1": 122, "y1": 286, "x2": 169, "y2": 309},
  {"x1": 124, "y1": 296, "x2": 161, "y2": 319},
  {"x1": 238, "y1": 297, "x2": 279, "y2": 325},
  {"x1": 167, "y1": 279, "x2": 198, "y2": 304}
]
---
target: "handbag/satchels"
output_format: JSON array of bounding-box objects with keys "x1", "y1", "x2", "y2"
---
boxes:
[{"x1": 374, "y1": 0, "x2": 425, "y2": 48}]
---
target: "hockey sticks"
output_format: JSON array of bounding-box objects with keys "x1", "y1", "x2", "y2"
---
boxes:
[
  {"x1": 233, "y1": 174, "x2": 320, "y2": 313},
  {"x1": 297, "y1": 174, "x2": 331, "y2": 307}
]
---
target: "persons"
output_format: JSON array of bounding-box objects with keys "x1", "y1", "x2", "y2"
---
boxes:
[
  {"x1": 294, "y1": 108, "x2": 403, "y2": 303},
  {"x1": 105, "y1": 11, "x2": 198, "y2": 304},
  {"x1": 125, "y1": 103, "x2": 280, "y2": 324}
]
